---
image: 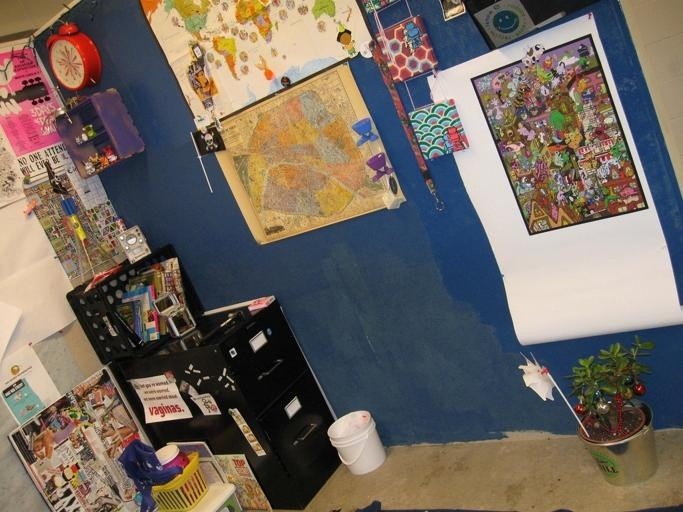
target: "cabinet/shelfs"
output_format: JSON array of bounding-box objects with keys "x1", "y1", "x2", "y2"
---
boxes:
[
  {"x1": 115, "y1": 295, "x2": 339, "y2": 512},
  {"x1": 50, "y1": 87, "x2": 146, "y2": 179}
]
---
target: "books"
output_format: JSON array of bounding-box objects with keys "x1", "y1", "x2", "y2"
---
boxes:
[{"x1": 106, "y1": 257, "x2": 187, "y2": 352}]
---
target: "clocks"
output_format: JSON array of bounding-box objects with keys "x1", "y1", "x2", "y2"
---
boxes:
[{"x1": 45, "y1": 19, "x2": 102, "y2": 97}]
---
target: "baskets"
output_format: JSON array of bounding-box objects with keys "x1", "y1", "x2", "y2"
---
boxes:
[{"x1": 150, "y1": 451, "x2": 208, "y2": 511}]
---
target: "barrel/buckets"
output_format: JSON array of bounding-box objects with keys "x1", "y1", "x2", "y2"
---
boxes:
[
  {"x1": 575, "y1": 398, "x2": 659, "y2": 487},
  {"x1": 325, "y1": 410, "x2": 387, "y2": 478}
]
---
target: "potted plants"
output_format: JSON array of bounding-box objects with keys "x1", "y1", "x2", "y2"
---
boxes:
[{"x1": 562, "y1": 335, "x2": 659, "y2": 488}]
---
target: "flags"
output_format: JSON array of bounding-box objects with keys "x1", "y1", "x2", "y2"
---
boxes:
[{"x1": 191, "y1": 127, "x2": 226, "y2": 157}]
